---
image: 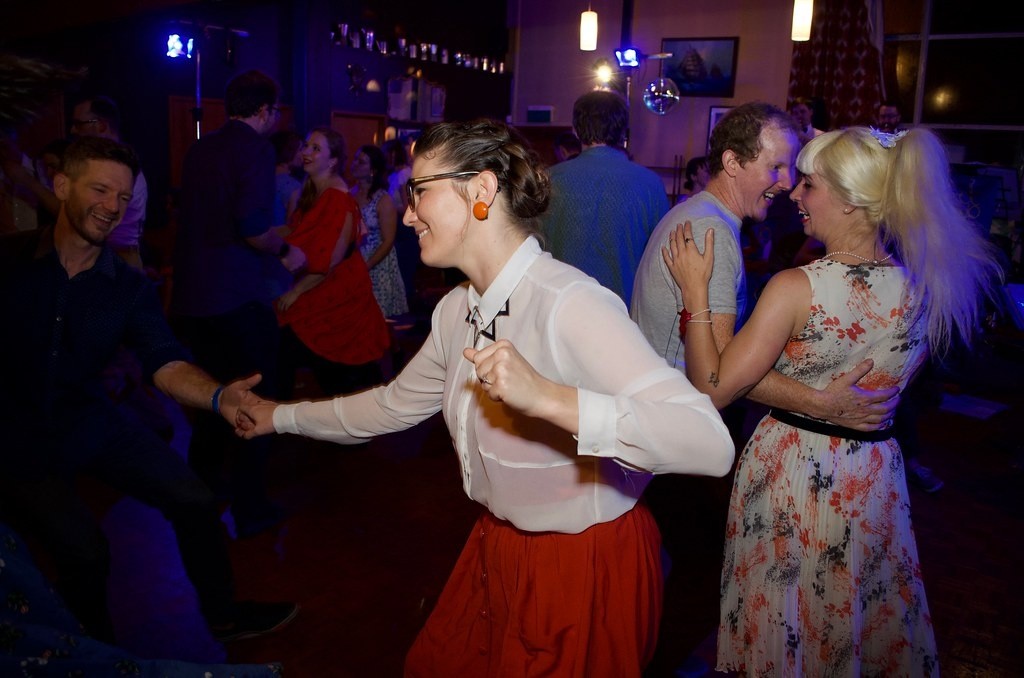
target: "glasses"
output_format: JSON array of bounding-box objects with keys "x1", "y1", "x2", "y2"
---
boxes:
[
  {"x1": 259, "y1": 104, "x2": 282, "y2": 121},
  {"x1": 405, "y1": 171, "x2": 501, "y2": 213}
]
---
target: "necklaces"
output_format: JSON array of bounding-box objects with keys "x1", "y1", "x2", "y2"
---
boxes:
[{"x1": 822, "y1": 251, "x2": 895, "y2": 266}]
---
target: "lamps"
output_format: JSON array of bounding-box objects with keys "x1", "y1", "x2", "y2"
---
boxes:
[{"x1": 347, "y1": 63, "x2": 381, "y2": 97}]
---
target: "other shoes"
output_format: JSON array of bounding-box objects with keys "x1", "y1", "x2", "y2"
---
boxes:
[
  {"x1": 904, "y1": 456, "x2": 945, "y2": 493},
  {"x1": 234, "y1": 500, "x2": 285, "y2": 535},
  {"x1": 211, "y1": 601, "x2": 299, "y2": 642}
]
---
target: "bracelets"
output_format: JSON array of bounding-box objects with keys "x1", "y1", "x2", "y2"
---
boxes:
[
  {"x1": 680, "y1": 308, "x2": 712, "y2": 330},
  {"x1": 212, "y1": 385, "x2": 224, "y2": 412}
]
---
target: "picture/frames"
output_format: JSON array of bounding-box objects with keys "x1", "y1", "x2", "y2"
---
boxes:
[
  {"x1": 705, "y1": 105, "x2": 737, "y2": 157},
  {"x1": 658, "y1": 36, "x2": 739, "y2": 98}
]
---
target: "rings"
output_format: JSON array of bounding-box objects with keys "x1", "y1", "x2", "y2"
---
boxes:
[{"x1": 482, "y1": 375, "x2": 493, "y2": 385}]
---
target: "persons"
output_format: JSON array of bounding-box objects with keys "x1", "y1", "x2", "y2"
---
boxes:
[{"x1": 0, "y1": 69, "x2": 1024, "y2": 678}]
